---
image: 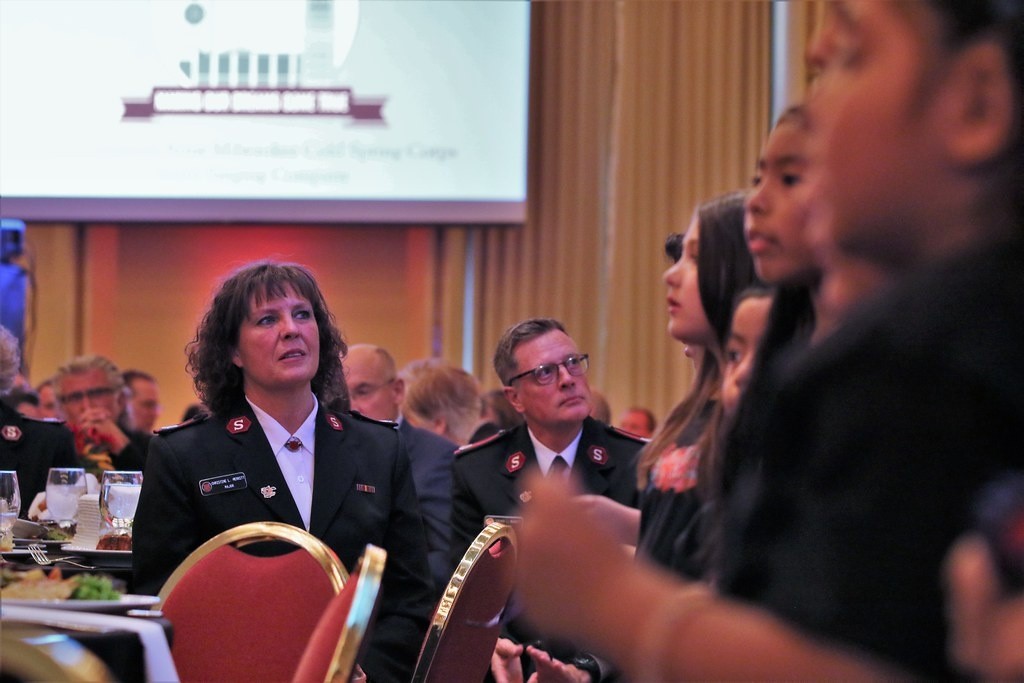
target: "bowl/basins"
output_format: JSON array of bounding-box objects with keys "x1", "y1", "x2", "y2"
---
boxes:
[{"x1": 10, "y1": 519, "x2": 49, "y2": 539}]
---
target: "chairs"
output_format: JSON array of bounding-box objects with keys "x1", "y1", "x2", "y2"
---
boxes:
[{"x1": 149, "y1": 521, "x2": 518, "y2": 683}]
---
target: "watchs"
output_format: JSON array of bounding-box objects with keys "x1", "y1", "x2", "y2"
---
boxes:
[{"x1": 567, "y1": 655, "x2": 600, "y2": 683}]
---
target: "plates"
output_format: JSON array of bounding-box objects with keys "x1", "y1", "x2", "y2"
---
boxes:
[
  {"x1": 14, "y1": 536, "x2": 71, "y2": 545},
  {"x1": 0, "y1": 549, "x2": 47, "y2": 561},
  {"x1": 63, "y1": 544, "x2": 133, "y2": 567}
]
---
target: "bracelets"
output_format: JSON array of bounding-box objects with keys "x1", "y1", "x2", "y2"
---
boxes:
[{"x1": 636, "y1": 583, "x2": 717, "y2": 683}]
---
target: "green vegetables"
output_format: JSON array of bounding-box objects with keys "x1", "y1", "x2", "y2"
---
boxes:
[{"x1": 73, "y1": 573, "x2": 122, "y2": 600}]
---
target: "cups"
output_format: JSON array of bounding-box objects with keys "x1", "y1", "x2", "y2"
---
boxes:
[{"x1": 99, "y1": 470, "x2": 144, "y2": 535}]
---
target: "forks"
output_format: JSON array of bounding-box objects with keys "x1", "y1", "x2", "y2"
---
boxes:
[{"x1": 27, "y1": 546, "x2": 96, "y2": 570}]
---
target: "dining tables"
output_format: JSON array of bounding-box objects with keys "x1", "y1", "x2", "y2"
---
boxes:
[{"x1": 0, "y1": 523, "x2": 173, "y2": 683}]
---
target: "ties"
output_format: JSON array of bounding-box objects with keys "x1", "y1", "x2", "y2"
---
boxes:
[{"x1": 551, "y1": 455, "x2": 566, "y2": 498}]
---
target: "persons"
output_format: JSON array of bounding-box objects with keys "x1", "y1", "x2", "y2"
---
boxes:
[{"x1": 0, "y1": 0, "x2": 1024, "y2": 683}]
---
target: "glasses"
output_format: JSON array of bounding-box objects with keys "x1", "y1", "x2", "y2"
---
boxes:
[
  {"x1": 508, "y1": 352, "x2": 590, "y2": 387},
  {"x1": 57, "y1": 383, "x2": 120, "y2": 404}
]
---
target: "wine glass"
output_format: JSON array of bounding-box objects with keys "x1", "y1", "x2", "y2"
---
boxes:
[
  {"x1": 0, "y1": 471, "x2": 20, "y2": 553},
  {"x1": 47, "y1": 468, "x2": 89, "y2": 540}
]
